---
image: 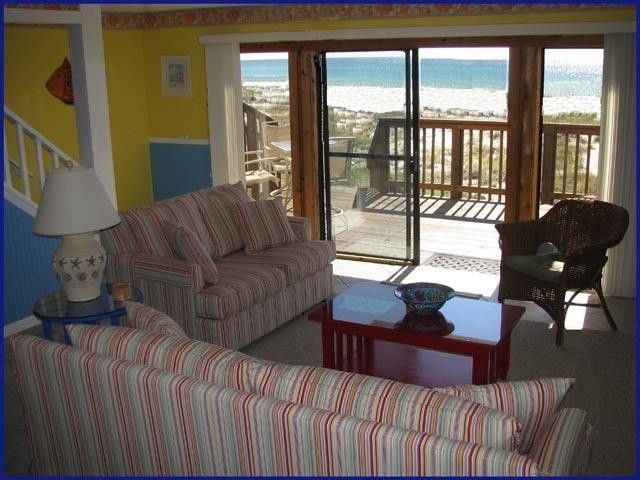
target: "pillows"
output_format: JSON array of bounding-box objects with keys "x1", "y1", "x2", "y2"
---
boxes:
[
  {"x1": 102, "y1": 181, "x2": 299, "y2": 284},
  {"x1": 124, "y1": 300, "x2": 189, "y2": 340},
  {"x1": 431, "y1": 376, "x2": 575, "y2": 448},
  {"x1": 230, "y1": 356, "x2": 523, "y2": 449},
  {"x1": 64, "y1": 323, "x2": 271, "y2": 398}
]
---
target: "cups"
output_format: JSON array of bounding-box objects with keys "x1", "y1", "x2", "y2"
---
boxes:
[{"x1": 111, "y1": 282, "x2": 132, "y2": 302}]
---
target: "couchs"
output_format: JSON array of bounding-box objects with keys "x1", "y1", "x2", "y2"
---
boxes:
[
  {"x1": 98, "y1": 182, "x2": 337, "y2": 352},
  {"x1": 5, "y1": 333, "x2": 594, "y2": 477}
]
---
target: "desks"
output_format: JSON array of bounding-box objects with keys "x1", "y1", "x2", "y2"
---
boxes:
[{"x1": 31, "y1": 284, "x2": 144, "y2": 346}]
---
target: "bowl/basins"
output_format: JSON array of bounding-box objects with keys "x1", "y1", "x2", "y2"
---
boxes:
[
  {"x1": 393, "y1": 281, "x2": 457, "y2": 314},
  {"x1": 393, "y1": 309, "x2": 455, "y2": 338}
]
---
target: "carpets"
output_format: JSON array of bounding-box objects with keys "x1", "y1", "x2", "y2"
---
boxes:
[{"x1": 420, "y1": 251, "x2": 501, "y2": 276}]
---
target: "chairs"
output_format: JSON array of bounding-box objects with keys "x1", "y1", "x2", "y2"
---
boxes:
[{"x1": 494, "y1": 197, "x2": 629, "y2": 347}]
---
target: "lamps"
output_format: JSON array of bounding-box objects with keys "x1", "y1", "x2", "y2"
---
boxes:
[{"x1": 31, "y1": 159, "x2": 122, "y2": 303}]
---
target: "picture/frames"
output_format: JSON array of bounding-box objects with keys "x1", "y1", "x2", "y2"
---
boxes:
[{"x1": 160, "y1": 54, "x2": 192, "y2": 97}]
